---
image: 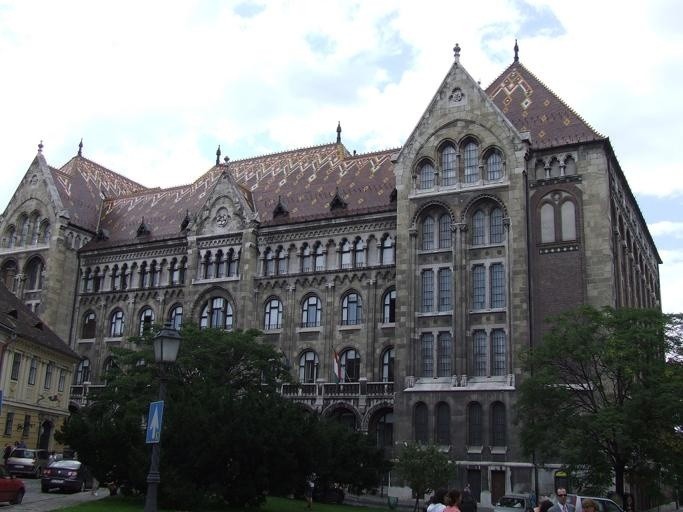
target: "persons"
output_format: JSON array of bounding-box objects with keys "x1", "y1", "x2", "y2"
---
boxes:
[
  {"x1": 3, "y1": 443, "x2": 12, "y2": 474},
  {"x1": 15, "y1": 439, "x2": 26, "y2": 448},
  {"x1": 49, "y1": 451, "x2": 57, "y2": 465},
  {"x1": 528, "y1": 487, "x2": 596, "y2": 512},
  {"x1": 338, "y1": 486, "x2": 345, "y2": 505},
  {"x1": 426, "y1": 483, "x2": 477, "y2": 512},
  {"x1": 303, "y1": 478, "x2": 315, "y2": 509}
]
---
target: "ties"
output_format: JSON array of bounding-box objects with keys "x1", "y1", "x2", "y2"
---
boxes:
[{"x1": 562, "y1": 504, "x2": 566, "y2": 512}]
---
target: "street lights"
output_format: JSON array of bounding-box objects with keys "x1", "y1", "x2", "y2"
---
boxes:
[{"x1": 144, "y1": 320, "x2": 183, "y2": 512}]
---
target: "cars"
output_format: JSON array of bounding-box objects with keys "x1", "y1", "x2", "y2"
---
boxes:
[
  {"x1": 0, "y1": 464, "x2": 26, "y2": 505},
  {"x1": 41, "y1": 459, "x2": 93, "y2": 494}
]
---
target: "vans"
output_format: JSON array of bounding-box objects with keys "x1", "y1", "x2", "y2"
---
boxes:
[
  {"x1": 492, "y1": 495, "x2": 532, "y2": 512},
  {"x1": 6, "y1": 447, "x2": 50, "y2": 479}
]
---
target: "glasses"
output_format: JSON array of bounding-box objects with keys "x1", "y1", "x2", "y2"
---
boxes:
[{"x1": 557, "y1": 494, "x2": 566, "y2": 497}]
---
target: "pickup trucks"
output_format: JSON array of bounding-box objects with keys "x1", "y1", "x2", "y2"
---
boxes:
[{"x1": 562, "y1": 493, "x2": 626, "y2": 512}]
